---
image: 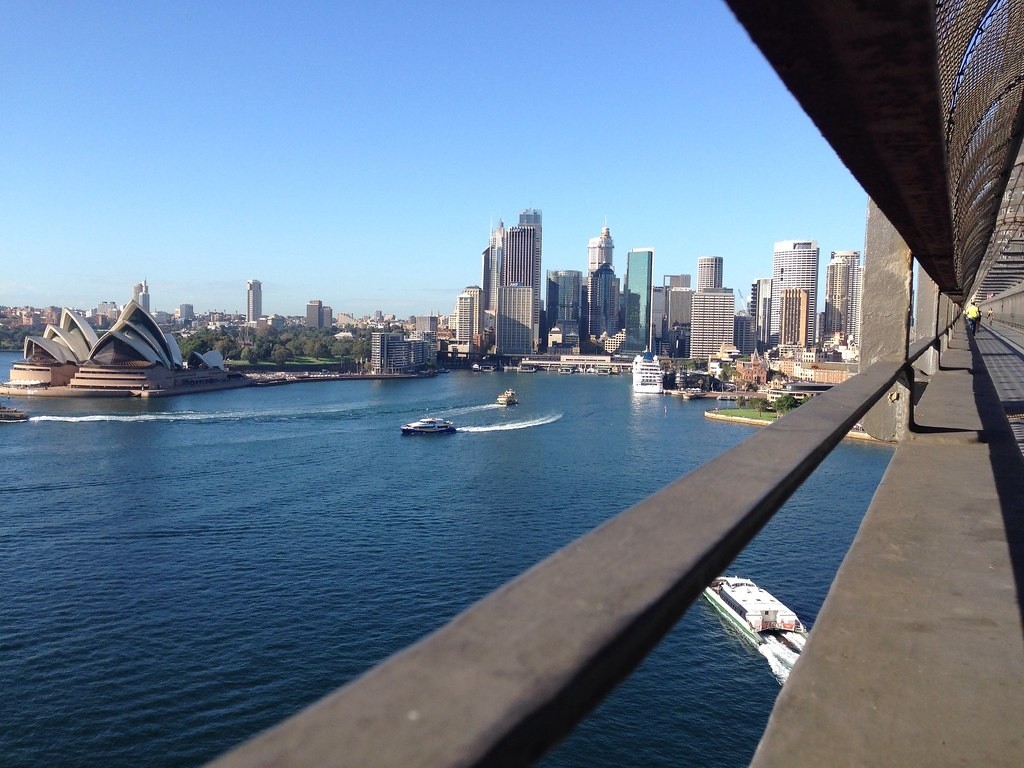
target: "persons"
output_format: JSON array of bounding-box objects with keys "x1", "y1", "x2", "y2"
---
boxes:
[
  {"x1": 976, "y1": 305, "x2": 982, "y2": 332},
  {"x1": 966, "y1": 301, "x2": 980, "y2": 336},
  {"x1": 987, "y1": 307, "x2": 996, "y2": 326},
  {"x1": 963, "y1": 305, "x2": 970, "y2": 322}
]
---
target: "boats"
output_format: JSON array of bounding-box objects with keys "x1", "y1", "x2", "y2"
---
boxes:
[
  {"x1": 401, "y1": 415, "x2": 456, "y2": 433},
  {"x1": 495, "y1": 389, "x2": 521, "y2": 407},
  {"x1": 0, "y1": 404, "x2": 27, "y2": 422},
  {"x1": 677, "y1": 386, "x2": 760, "y2": 402},
  {"x1": 707, "y1": 575, "x2": 808, "y2": 648},
  {"x1": 423, "y1": 363, "x2": 629, "y2": 377}
]
